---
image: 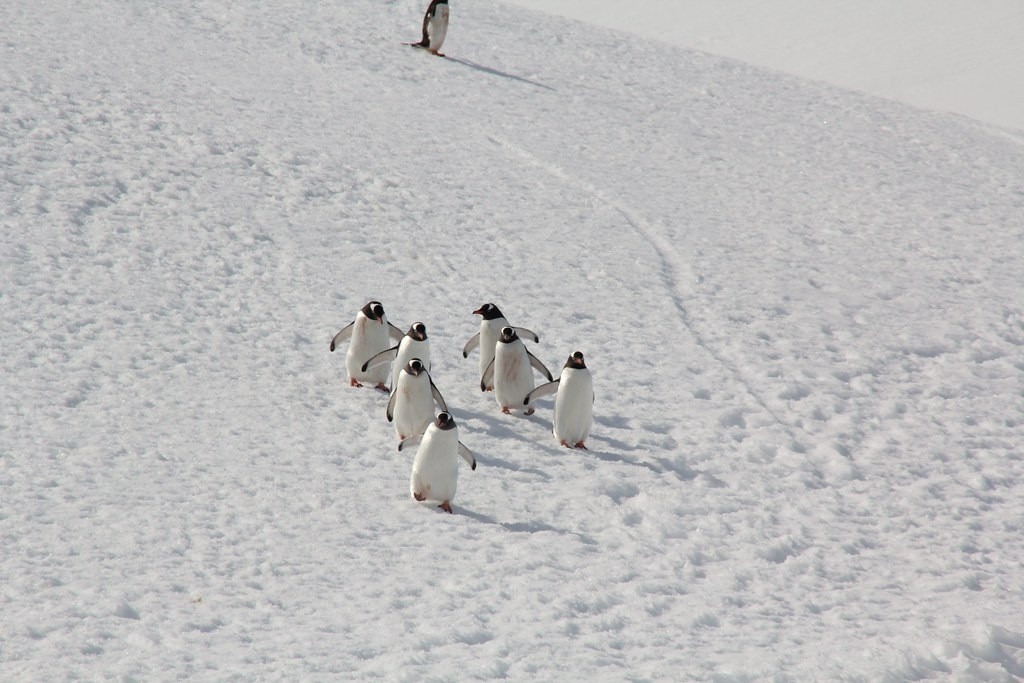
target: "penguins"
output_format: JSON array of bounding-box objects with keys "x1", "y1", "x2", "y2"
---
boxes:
[
  {"x1": 329, "y1": 300, "x2": 477, "y2": 514},
  {"x1": 400, "y1": 0, "x2": 450, "y2": 57},
  {"x1": 462, "y1": 302, "x2": 596, "y2": 451}
]
